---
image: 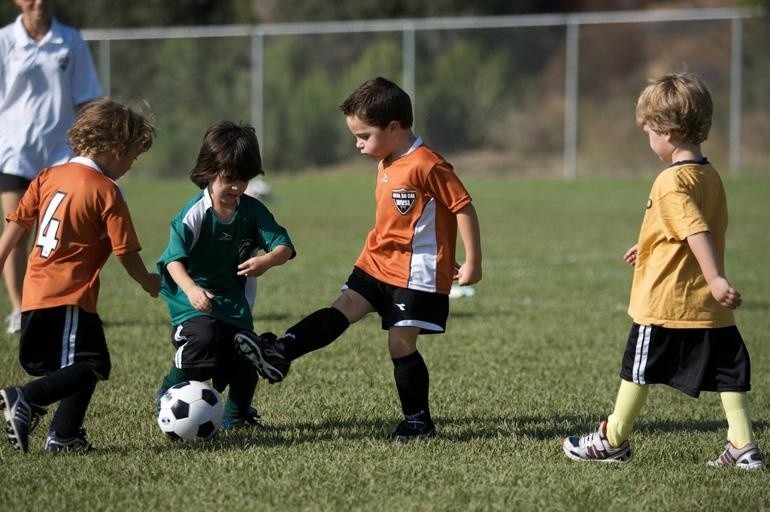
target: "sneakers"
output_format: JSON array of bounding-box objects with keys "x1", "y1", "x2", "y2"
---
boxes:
[
  {"x1": 45, "y1": 427, "x2": 95, "y2": 455},
  {"x1": 233, "y1": 330, "x2": 291, "y2": 384},
  {"x1": 222, "y1": 416, "x2": 266, "y2": 434},
  {"x1": 8, "y1": 311, "x2": 21, "y2": 334},
  {"x1": 705, "y1": 442, "x2": 763, "y2": 471},
  {"x1": 562, "y1": 421, "x2": 632, "y2": 464},
  {"x1": 0, "y1": 386, "x2": 47, "y2": 455},
  {"x1": 386, "y1": 420, "x2": 436, "y2": 443}
]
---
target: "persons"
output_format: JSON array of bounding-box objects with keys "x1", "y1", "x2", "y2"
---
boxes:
[
  {"x1": 561, "y1": 75, "x2": 766, "y2": 472},
  {"x1": 152, "y1": 119, "x2": 298, "y2": 421},
  {"x1": 0, "y1": 2, "x2": 101, "y2": 333},
  {"x1": 233, "y1": 80, "x2": 483, "y2": 442},
  {"x1": 0, "y1": 100, "x2": 163, "y2": 456}
]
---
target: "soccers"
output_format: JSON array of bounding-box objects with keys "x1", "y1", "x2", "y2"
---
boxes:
[{"x1": 154, "y1": 380, "x2": 224, "y2": 442}]
